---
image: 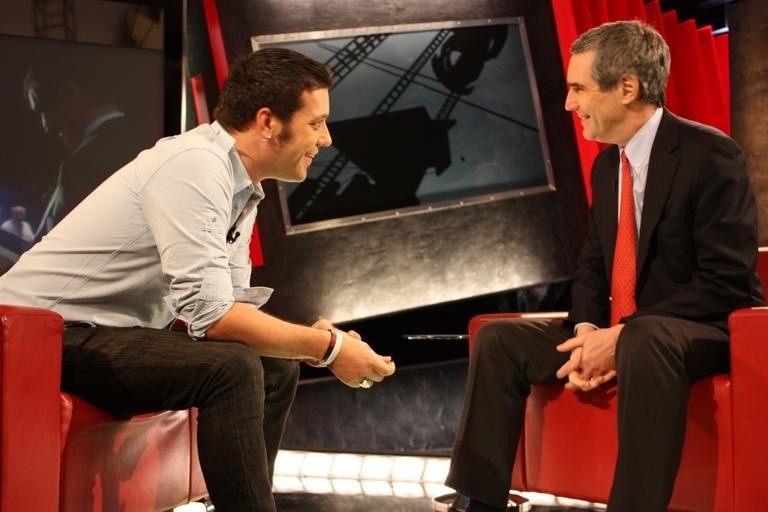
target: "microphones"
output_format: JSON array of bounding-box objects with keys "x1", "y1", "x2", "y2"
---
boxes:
[{"x1": 232, "y1": 232, "x2": 241, "y2": 243}]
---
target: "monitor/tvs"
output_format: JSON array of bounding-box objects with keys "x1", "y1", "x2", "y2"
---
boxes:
[
  {"x1": 0, "y1": 0, "x2": 189, "y2": 276},
  {"x1": 251, "y1": 15, "x2": 557, "y2": 234}
]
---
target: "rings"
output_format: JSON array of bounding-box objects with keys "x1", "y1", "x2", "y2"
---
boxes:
[{"x1": 359, "y1": 380, "x2": 371, "y2": 390}]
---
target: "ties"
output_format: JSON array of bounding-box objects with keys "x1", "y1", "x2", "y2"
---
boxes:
[{"x1": 610, "y1": 151, "x2": 638, "y2": 328}]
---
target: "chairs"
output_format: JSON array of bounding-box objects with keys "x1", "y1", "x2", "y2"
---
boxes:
[
  {"x1": 468, "y1": 248, "x2": 768, "y2": 512},
  {"x1": 0, "y1": 302, "x2": 212, "y2": 512}
]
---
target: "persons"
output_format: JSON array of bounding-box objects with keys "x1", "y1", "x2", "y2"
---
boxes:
[
  {"x1": 22, "y1": 44, "x2": 144, "y2": 248},
  {"x1": 0, "y1": 45, "x2": 397, "y2": 512},
  {"x1": 2, "y1": 203, "x2": 36, "y2": 243},
  {"x1": 445, "y1": 19, "x2": 766, "y2": 510}
]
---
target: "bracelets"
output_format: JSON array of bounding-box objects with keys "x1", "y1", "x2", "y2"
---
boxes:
[
  {"x1": 314, "y1": 330, "x2": 342, "y2": 368},
  {"x1": 321, "y1": 328, "x2": 336, "y2": 363}
]
